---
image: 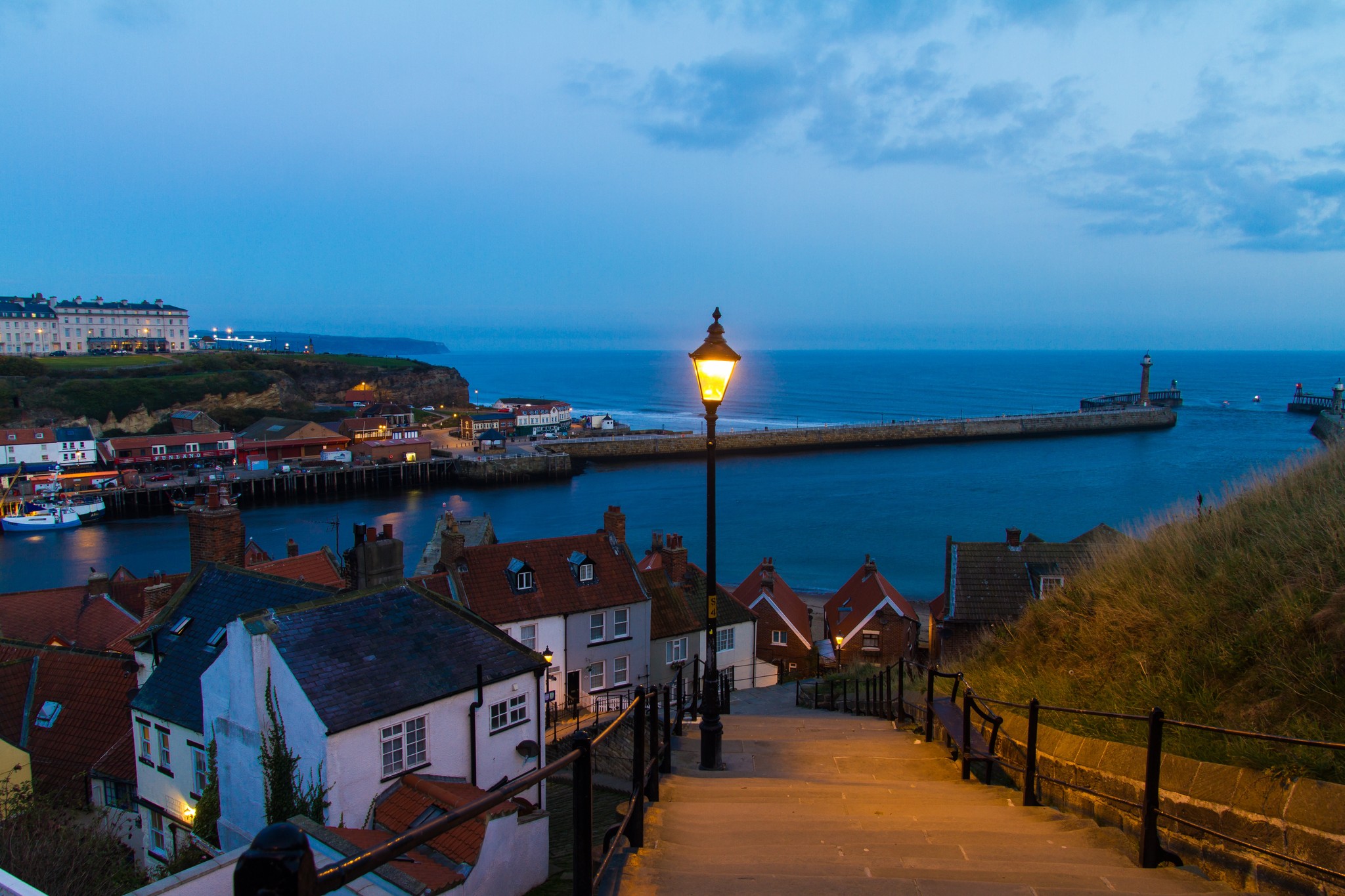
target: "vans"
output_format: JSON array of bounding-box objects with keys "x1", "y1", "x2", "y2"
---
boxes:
[
  {"x1": 353, "y1": 402, "x2": 365, "y2": 408},
  {"x1": 543, "y1": 433, "x2": 558, "y2": 440}
]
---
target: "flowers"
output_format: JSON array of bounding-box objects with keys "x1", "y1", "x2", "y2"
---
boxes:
[
  {"x1": 584, "y1": 666, "x2": 594, "y2": 674},
  {"x1": 670, "y1": 660, "x2": 685, "y2": 671}
]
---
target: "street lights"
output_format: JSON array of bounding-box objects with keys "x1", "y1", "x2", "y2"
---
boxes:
[
  {"x1": 686, "y1": 306, "x2": 743, "y2": 773},
  {"x1": 592, "y1": 426, "x2": 594, "y2": 440},
  {"x1": 541, "y1": 645, "x2": 554, "y2": 729},
  {"x1": 274, "y1": 336, "x2": 277, "y2": 350},
  {"x1": 701, "y1": 421, "x2": 703, "y2": 435},
  {"x1": 453, "y1": 411, "x2": 460, "y2": 440},
  {"x1": 475, "y1": 389, "x2": 478, "y2": 413},
  {"x1": 882, "y1": 412, "x2": 883, "y2": 424},
  {"x1": 305, "y1": 345, "x2": 308, "y2": 363},
  {"x1": 796, "y1": 416, "x2": 799, "y2": 429},
  {"x1": 39, "y1": 328, "x2": 45, "y2": 357},
  {"x1": 540, "y1": 395, "x2": 544, "y2": 405},
  {"x1": 835, "y1": 630, "x2": 844, "y2": 674},
  {"x1": 483, "y1": 442, "x2": 485, "y2": 457},
  {"x1": 145, "y1": 328, "x2": 150, "y2": 355},
  {"x1": 960, "y1": 408, "x2": 963, "y2": 420},
  {"x1": 212, "y1": 327, "x2": 220, "y2": 351},
  {"x1": 1031, "y1": 405, "x2": 1033, "y2": 416},
  {"x1": 440, "y1": 404, "x2": 444, "y2": 430},
  {"x1": 228, "y1": 328, "x2": 233, "y2": 348}
]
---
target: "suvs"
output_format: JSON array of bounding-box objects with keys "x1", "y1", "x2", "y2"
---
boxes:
[{"x1": 49, "y1": 350, "x2": 68, "y2": 357}]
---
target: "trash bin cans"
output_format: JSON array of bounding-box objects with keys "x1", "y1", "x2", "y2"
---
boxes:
[
  {"x1": 356, "y1": 458, "x2": 360, "y2": 466},
  {"x1": 187, "y1": 469, "x2": 196, "y2": 476}
]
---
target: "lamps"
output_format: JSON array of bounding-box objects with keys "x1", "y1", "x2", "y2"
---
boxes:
[{"x1": 184, "y1": 808, "x2": 193, "y2": 820}]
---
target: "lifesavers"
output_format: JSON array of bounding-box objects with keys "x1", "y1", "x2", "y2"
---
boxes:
[
  {"x1": 390, "y1": 455, "x2": 393, "y2": 458},
  {"x1": 54, "y1": 515, "x2": 59, "y2": 521}
]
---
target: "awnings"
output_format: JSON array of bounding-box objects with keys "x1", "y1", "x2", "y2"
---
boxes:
[{"x1": 474, "y1": 428, "x2": 498, "y2": 433}]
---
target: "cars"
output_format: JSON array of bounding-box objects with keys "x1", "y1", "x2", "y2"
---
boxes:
[
  {"x1": 407, "y1": 405, "x2": 415, "y2": 409},
  {"x1": 93, "y1": 349, "x2": 128, "y2": 355},
  {"x1": 422, "y1": 406, "x2": 435, "y2": 411},
  {"x1": 497, "y1": 408, "x2": 509, "y2": 413},
  {"x1": 150, "y1": 472, "x2": 175, "y2": 481}
]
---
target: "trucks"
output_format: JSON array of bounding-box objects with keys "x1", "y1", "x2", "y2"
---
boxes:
[{"x1": 274, "y1": 464, "x2": 290, "y2": 473}]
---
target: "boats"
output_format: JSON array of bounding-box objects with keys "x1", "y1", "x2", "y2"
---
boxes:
[
  {"x1": 168, "y1": 492, "x2": 242, "y2": 510},
  {"x1": 1252, "y1": 395, "x2": 1261, "y2": 403},
  {"x1": 0, "y1": 466, "x2": 107, "y2": 534},
  {"x1": 1220, "y1": 401, "x2": 1230, "y2": 410}
]
